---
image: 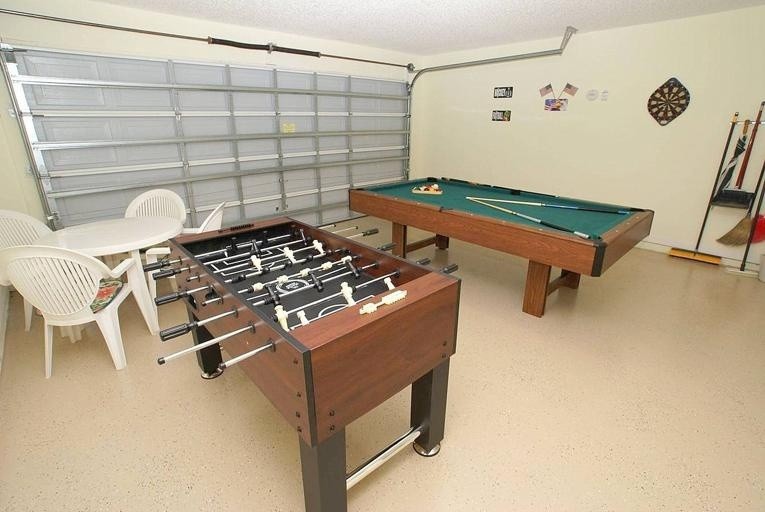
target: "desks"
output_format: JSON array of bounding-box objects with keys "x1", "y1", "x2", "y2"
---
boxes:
[
  {"x1": 349, "y1": 177, "x2": 655, "y2": 317},
  {"x1": 30, "y1": 215, "x2": 185, "y2": 339}
]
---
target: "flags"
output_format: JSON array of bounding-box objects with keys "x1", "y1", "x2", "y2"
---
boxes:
[
  {"x1": 539, "y1": 83, "x2": 553, "y2": 96},
  {"x1": 563, "y1": 82, "x2": 579, "y2": 96}
]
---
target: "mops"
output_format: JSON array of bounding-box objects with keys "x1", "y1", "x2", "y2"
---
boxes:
[{"x1": 668, "y1": 111, "x2": 739, "y2": 266}]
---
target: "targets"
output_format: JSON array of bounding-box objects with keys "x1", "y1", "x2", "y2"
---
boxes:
[{"x1": 648, "y1": 78, "x2": 690, "y2": 127}]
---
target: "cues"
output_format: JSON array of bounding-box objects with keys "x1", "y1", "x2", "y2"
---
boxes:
[
  {"x1": 468, "y1": 199, "x2": 591, "y2": 240},
  {"x1": 466, "y1": 196, "x2": 630, "y2": 215}
]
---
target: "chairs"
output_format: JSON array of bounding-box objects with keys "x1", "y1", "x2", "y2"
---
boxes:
[
  {"x1": 144, "y1": 201, "x2": 224, "y2": 316},
  {"x1": 1, "y1": 206, "x2": 55, "y2": 353},
  {"x1": 112, "y1": 189, "x2": 187, "y2": 269},
  {"x1": 2, "y1": 245, "x2": 159, "y2": 381}
]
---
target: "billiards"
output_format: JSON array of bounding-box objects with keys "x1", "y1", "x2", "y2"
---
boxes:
[{"x1": 413, "y1": 183, "x2": 441, "y2": 191}]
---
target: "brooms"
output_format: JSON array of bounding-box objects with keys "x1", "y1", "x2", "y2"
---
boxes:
[{"x1": 715, "y1": 158, "x2": 764, "y2": 247}]
